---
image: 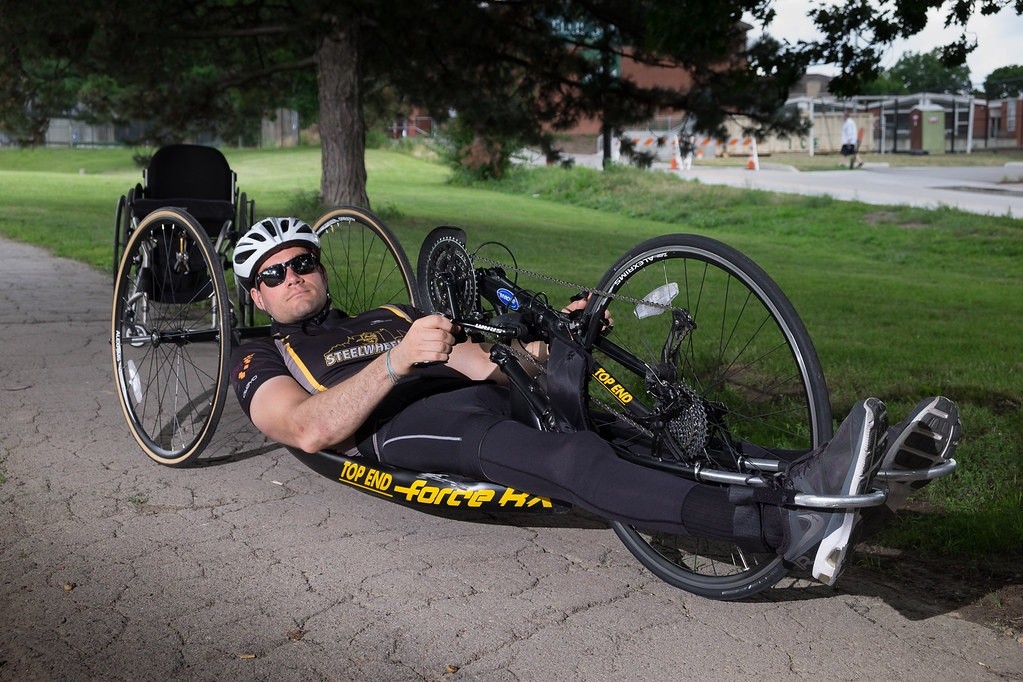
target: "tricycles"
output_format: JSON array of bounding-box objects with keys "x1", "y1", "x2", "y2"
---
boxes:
[{"x1": 108, "y1": 202, "x2": 961, "y2": 605}]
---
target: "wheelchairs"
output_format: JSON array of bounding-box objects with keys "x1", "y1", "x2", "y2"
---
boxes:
[{"x1": 109, "y1": 143, "x2": 256, "y2": 346}]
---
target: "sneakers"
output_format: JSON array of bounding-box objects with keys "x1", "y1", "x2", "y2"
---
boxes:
[
  {"x1": 858, "y1": 396, "x2": 963, "y2": 543},
  {"x1": 776, "y1": 397, "x2": 890, "y2": 587}
]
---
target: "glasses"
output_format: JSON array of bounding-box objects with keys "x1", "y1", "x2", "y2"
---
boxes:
[{"x1": 255, "y1": 254, "x2": 319, "y2": 292}]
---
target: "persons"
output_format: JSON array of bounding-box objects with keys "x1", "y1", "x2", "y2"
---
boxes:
[
  {"x1": 838, "y1": 108, "x2": 862, "y2": 169},
  {"x1": 226, "y1": 217, "x2": 958, "y2": 589}
]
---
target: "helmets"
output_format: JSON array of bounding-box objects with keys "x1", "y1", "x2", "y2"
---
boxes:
[{"x1": 232, "y1": 217, "x2": 321, "y2": 293}]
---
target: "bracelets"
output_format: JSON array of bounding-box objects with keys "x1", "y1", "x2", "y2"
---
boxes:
[{"x1": 385, "y1": 347, "x2": 399, "y2": 386}]
